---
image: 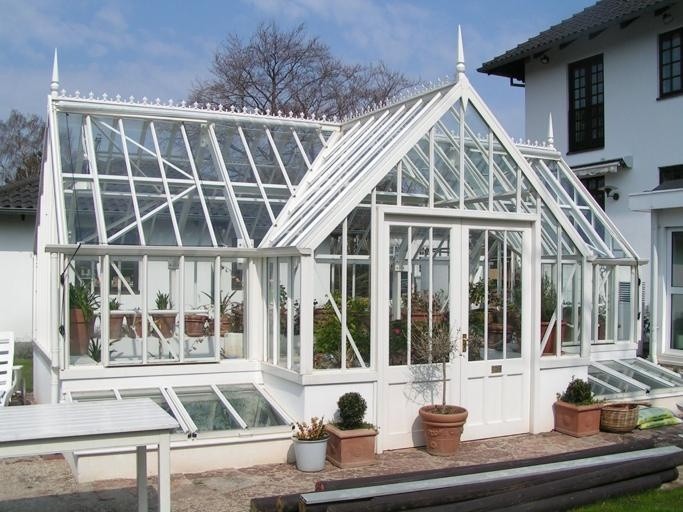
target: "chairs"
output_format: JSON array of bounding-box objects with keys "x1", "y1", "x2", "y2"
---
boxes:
[{"x1": 0, "y1": 331, "x2": 24, "y2": 406}]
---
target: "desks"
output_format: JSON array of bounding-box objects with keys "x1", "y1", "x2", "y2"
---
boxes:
[{"x1": 1, "y1": 397, "x2": 180, "y2": 512}]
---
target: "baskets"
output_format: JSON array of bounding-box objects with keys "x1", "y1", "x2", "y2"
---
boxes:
[{"x1": 601, "y1": 405, "x2": 639, "y2": 432}]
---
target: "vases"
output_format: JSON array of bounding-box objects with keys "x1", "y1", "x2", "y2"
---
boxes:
[{"x1": 419, "y1": 401, "x2": 468, "y2": 457}]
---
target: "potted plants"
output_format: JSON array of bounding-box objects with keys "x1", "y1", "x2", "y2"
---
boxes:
[
  {"x1": 290, "y1": 392, "x2": 386, "y2": 473},
  {"x1": 400, "y1": 272, "x2": 567, "y2": 356},
  {"x1": 551, "y1": 375, "x2": 609, "y2": 439},
  {"x1": 67, "y1": 276, "x2": 245, "y2": 358}
]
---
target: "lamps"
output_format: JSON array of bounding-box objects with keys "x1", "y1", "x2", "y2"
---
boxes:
[{"x1": 598, "y1": 184, "x2": 619, "y2": 200}]
